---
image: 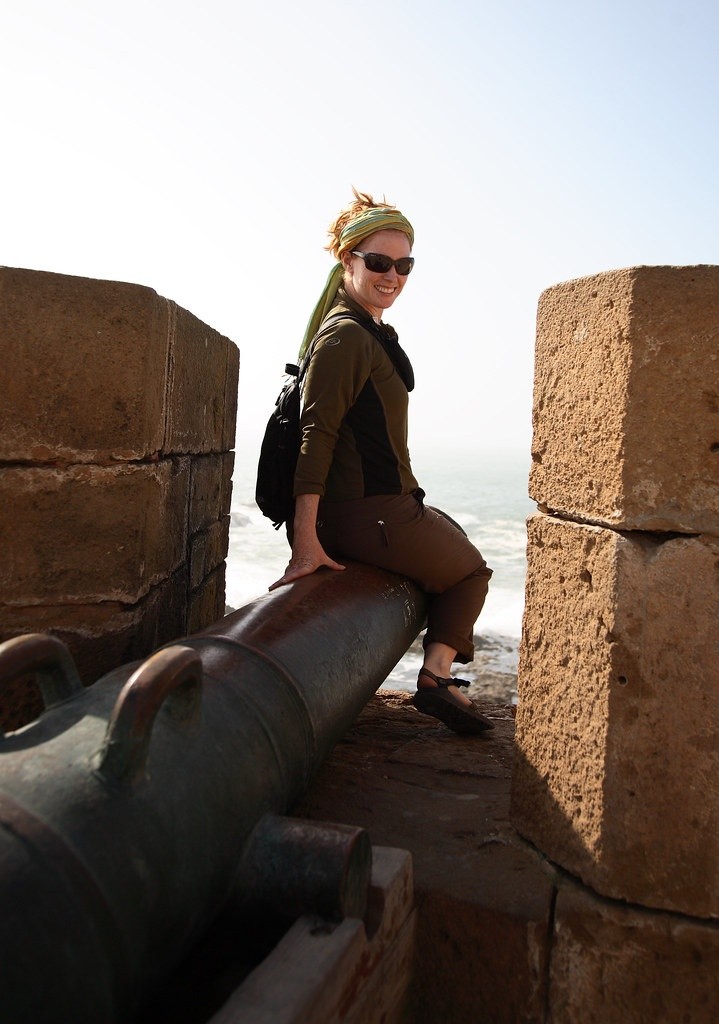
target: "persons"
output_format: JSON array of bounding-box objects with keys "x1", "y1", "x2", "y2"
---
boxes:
[{"x1": 269, "y1": 186, "x2": 495, "y2": 732}]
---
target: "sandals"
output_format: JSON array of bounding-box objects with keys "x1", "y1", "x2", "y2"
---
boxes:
[{"x1": 413, "y1": 667, "x2": 496, "y2": 733}]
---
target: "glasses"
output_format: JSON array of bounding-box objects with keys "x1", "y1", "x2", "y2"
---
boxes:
[{"x1": 350, "y1": 251, "x2": 415, "y2": 276}]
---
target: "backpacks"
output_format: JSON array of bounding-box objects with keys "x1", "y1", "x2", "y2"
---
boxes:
[{"x1": 255, "y1": 312, "x2": 378, "y2": 530}]
